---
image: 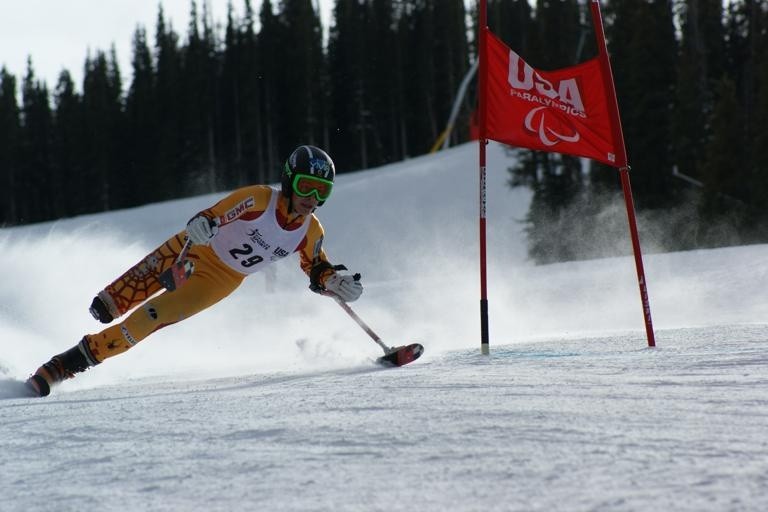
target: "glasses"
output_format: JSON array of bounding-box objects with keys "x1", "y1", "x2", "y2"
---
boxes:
[{"x1": 283, "y1": 170, "x2": 334, "y2": 202}]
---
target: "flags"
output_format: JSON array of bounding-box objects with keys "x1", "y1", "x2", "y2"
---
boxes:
[{"x1": 483, "y1": 28, "x2": 626, "y2": 168}]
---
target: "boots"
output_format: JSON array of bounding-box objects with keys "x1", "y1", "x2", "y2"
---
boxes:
[{"x1": 29, "y1": 357, "x2": 65, "y2": 396}]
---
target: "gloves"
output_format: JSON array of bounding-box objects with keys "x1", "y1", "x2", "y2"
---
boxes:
[
  {"x1": 326, "y1": 274, "x2": 363, "y2": 302},
  {"x1": 186, "y1": 214, "x2": 219, "y2": 247}
]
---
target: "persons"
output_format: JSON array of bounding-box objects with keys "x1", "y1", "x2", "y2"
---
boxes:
[{"x1": 22, "y1": 143, "x2": 364, "y2": 400}]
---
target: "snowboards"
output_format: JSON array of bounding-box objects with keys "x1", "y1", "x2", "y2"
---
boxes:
[{"x1": 26, "y1": 375, "x2": 50, "y2": 397}]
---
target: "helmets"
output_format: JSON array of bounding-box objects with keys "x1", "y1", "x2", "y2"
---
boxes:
[{"x1": 281, "y1": 145, "x2": 336, "y2": 206}]
